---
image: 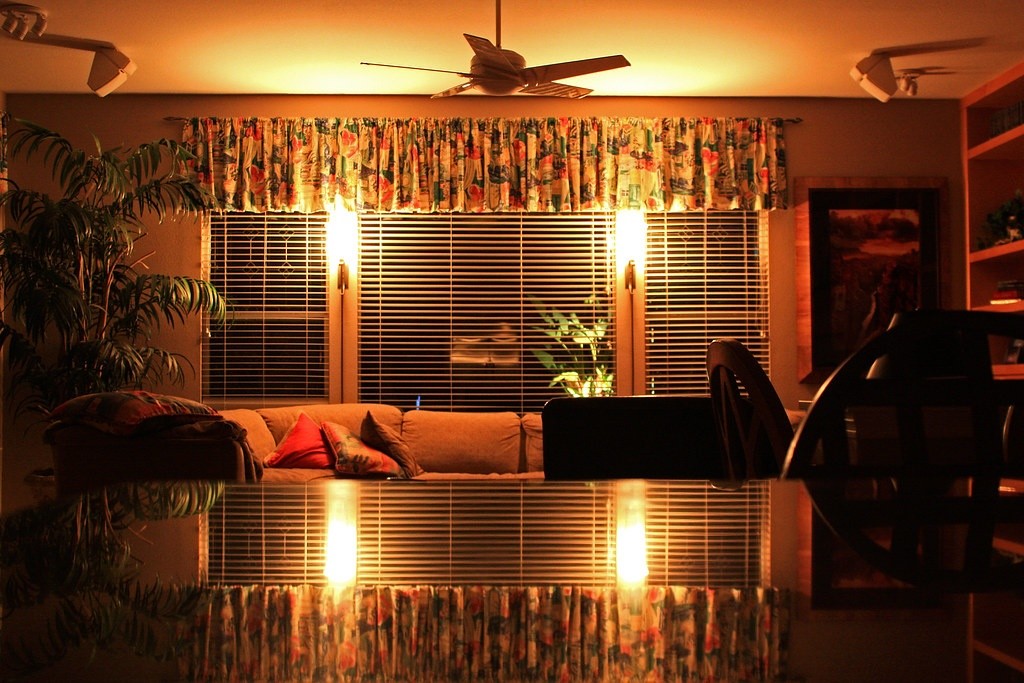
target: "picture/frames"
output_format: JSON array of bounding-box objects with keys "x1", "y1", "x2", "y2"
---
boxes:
[{"x1": 791, "y1": 175, "x2": 954, "y2": 383}]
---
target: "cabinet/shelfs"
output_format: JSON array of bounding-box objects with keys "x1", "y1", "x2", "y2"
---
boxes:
[{"x1": 960, "y1": 60, "x2": 1024, "y2": 683}]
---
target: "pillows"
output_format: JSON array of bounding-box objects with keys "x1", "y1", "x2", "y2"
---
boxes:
[
  {"x1": 43, "y1": 391, "x2": 224, "y2": 435},
  {"x1": 359, "y1": 410, "x2": 423, "y2": 478},
  {"x1": 262, "y1": 410, "x2": 336, "y2": 469},
  {"x1": 321, "y1": 421, "x2": 405, "y2": 478}
]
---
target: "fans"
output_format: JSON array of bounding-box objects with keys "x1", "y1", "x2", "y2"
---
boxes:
[{"x1": 360, "y1": 0, "x2": 632, "y2": 101}]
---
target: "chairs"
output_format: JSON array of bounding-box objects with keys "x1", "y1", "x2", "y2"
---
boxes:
[
  {"x1": 706, "y1": 338, "x2": 794, "y2": 480},
  {"x1": 779, "y1": 309, "x2": 1005, "y2": 478},
  {"x1": 541, "y1": 395, "x2": 780, "y2": 479}
]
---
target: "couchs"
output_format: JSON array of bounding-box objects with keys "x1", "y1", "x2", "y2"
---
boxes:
[{"x1": 42, "y1": 402, "x2": 543, "y2": 501}]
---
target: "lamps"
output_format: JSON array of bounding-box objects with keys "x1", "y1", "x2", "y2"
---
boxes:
[
  {"x1": 337, "y1": 259, "x2": 350, "y2": 403},
  {"x1": 1, "y1": 5, "x2": 49, "y2": 40},
  {"x1": 894, "y1": 70, "x2": 922, "y2": 96},
  {"x1": 85, "y1": 49, "x2": 138, "y2": 99},
  {"x1": 624, "y1": 260, "x2": 637, "y2": 394},
  {"x1": 851, "y1": 53, "x2": 899, "y2": 103}
]
